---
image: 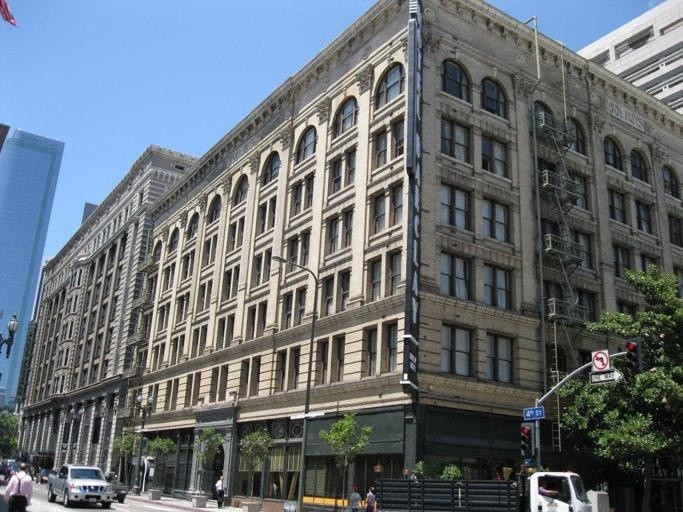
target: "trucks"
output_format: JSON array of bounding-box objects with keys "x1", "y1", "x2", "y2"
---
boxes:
[{"x1": 376, "y1": 471, "x2": 592, "y2": 512}]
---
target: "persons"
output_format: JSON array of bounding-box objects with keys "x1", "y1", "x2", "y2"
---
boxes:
[
  {"x1": 365, "y1": 486, "x2": 375, "y2": 512},
  {"x1": 539, "y1": 479, "x2": 559, "y2": 497},
  {"x1": 215, "y1": 476, "x2": 225, "y2": 509},
  {"x1": 350, "y1": 486, "x2": 363, "y2": 512},
  {"x1": 3, "y1": 463, "x2": 32, "y2": 512}
]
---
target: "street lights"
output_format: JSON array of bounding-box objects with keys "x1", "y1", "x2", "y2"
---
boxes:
[
  {"x1": 133, "y1": 395, "x2": 155, "y2": 496},
  {"x1": 272, "y1": 256, "x2": 318, "y2": 512}
]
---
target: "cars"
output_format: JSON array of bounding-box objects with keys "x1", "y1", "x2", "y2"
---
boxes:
[{"x1": 0, "y1": 460, "x2": 55, "y2": 483}]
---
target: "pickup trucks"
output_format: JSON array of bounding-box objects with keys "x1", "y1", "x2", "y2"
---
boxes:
[{"x1": 48, "y1": 463, "x2": 114, "y2": 510}]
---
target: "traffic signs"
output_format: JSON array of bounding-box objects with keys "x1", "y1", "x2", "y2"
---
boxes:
[
  {"x1": 524, "y1": 407, "x2": 545, "y2": 420},
  {"x1": 590, "y1": 369, "x2": 622, "y2": 384}
]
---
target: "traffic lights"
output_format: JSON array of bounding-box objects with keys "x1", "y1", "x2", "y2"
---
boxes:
[
  {"x1": 521, "y1": 423, "x2": 535, "y2": 457},
  {"x1": 627, "y1": 339, "x2": 642, "y2": 375}
]
